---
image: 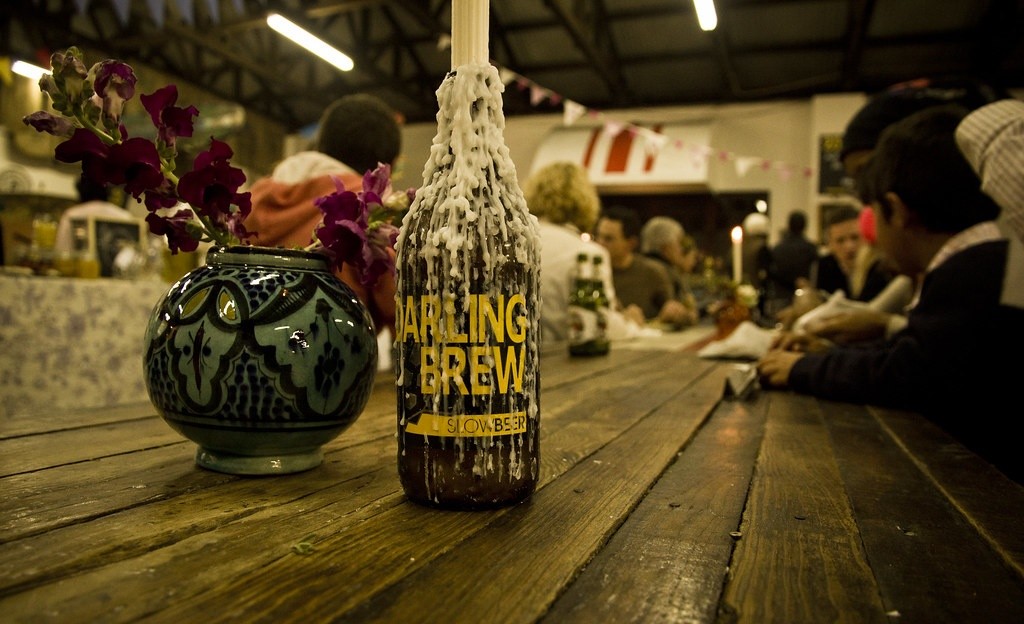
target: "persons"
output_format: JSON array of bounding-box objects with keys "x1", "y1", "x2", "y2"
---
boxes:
[
  {"x1": 234, "y1": 93, "x2": 720, "y2": 354},
  {"x1": 740, "y1": 89, "x2": 1024, "y2": 454}
]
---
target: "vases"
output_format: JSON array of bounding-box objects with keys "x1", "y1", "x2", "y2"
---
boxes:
[{"x1": 142, "y1": 249, "x2": 379, "y2": 476}]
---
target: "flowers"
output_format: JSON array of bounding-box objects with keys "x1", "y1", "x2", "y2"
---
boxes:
[{"x1": 22, "y1": 48, "x2": 414, "y2": 283}]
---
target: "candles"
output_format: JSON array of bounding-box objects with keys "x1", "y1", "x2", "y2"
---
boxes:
[
  {"x1": 731, "y1": 226, "x2": 743, "y2": 283},
  {"x1": 450, "y1": 0, "x2": 489, "y2": 71}
]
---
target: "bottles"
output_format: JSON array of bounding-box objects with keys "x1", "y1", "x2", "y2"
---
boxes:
[
  {"x1": 567, "y1": 253, "x2": 612, "y2": 359},
  {"x1": 394, "y1": 64, "x2": 543, "y2": 514}
]
